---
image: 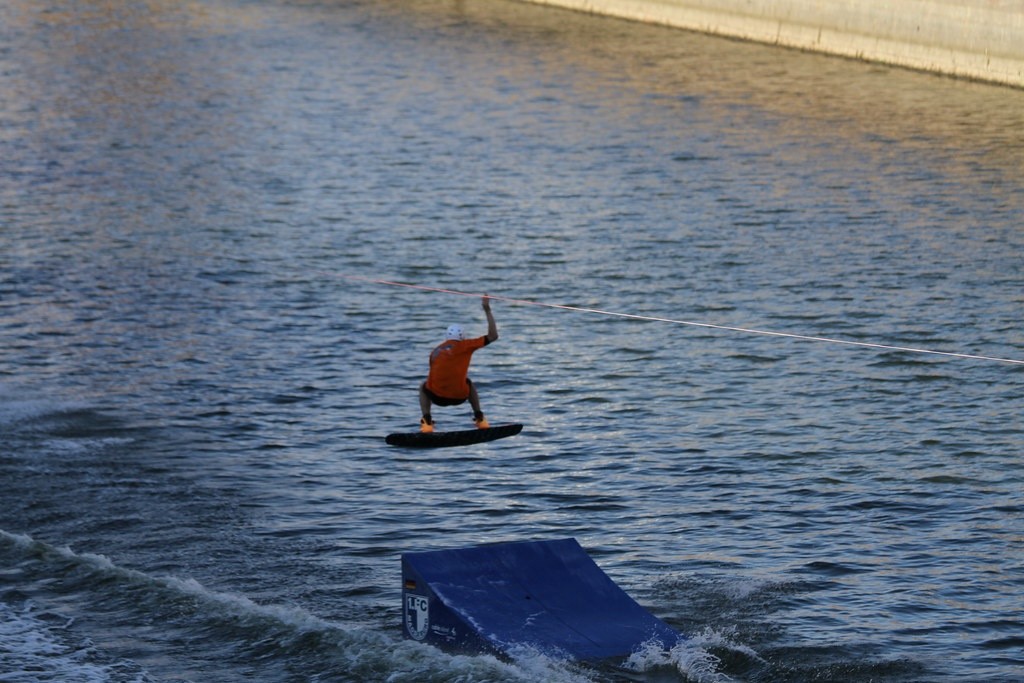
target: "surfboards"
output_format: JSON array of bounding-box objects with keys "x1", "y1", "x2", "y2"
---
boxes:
[{"x1": 385, "y1": 423, "x2": 524, "y2": 449}]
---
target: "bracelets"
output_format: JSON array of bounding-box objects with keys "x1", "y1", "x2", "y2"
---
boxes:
[{"x1": 483, "y1": 305, "x2": 490, "y2": 313}]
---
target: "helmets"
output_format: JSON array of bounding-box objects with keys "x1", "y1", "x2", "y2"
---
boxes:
[{"x1": 446, "y1": 324, "x2": 465, "y2": 340}]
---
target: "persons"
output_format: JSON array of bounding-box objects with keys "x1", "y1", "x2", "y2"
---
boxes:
[{"x1": 419, "y1": 293, "x2": 499, "y2": 431}]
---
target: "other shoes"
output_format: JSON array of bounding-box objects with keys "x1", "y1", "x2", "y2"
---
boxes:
[
  {"x1": 420, "y1": 418, "x2": 434, "y2": 432},
  {"x1": 476, "y1": 417, "x2": 489, "y2": 429}
]
